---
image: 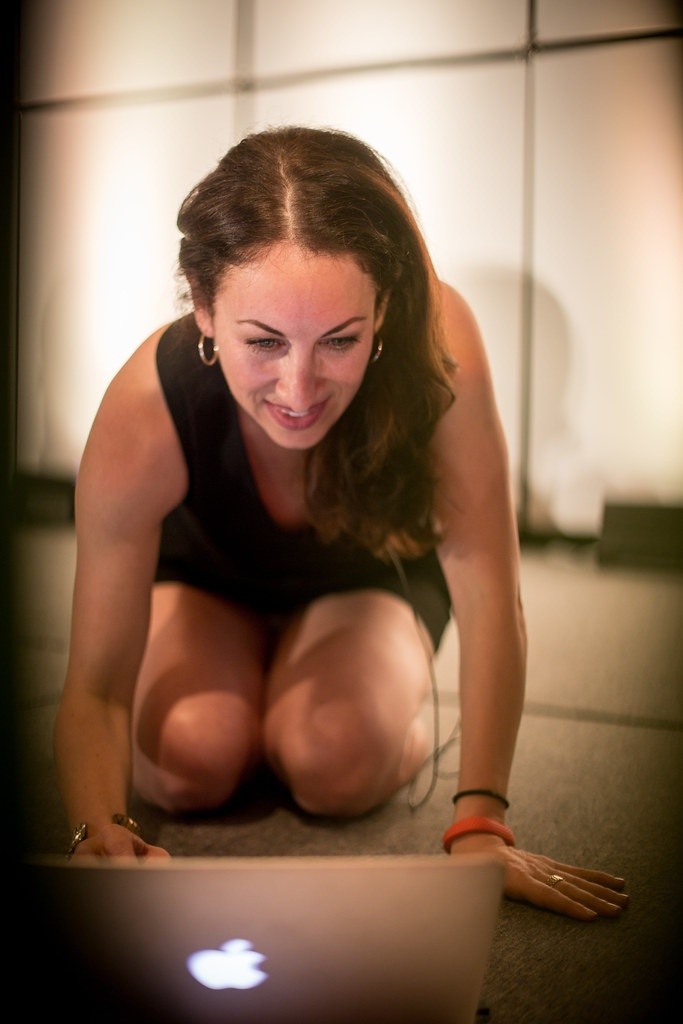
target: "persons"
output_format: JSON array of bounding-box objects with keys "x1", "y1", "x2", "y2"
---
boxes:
[{"x1": 52, "y1": 127, "x2": 629, "y2": 922}]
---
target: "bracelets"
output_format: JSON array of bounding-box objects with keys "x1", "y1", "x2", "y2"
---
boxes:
[
  {"x1": 452, "y1": 787, "x2": 510, "y2": 809},
  {"x1": 66, "y1": 813, "x2": 142, "y2": 862},
  {"x1": 442, "y1": 816, "x2": 515, "y2": 854}
]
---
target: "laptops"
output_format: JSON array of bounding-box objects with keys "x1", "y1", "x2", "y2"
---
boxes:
[{"x1": 22, "y1": 852, "x2": 502, "y2": 1024}]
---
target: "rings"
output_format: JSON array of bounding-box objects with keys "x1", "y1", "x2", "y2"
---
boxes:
[{"x1": 545, "y1": 873, "x2": 565, "y2": 889}]
